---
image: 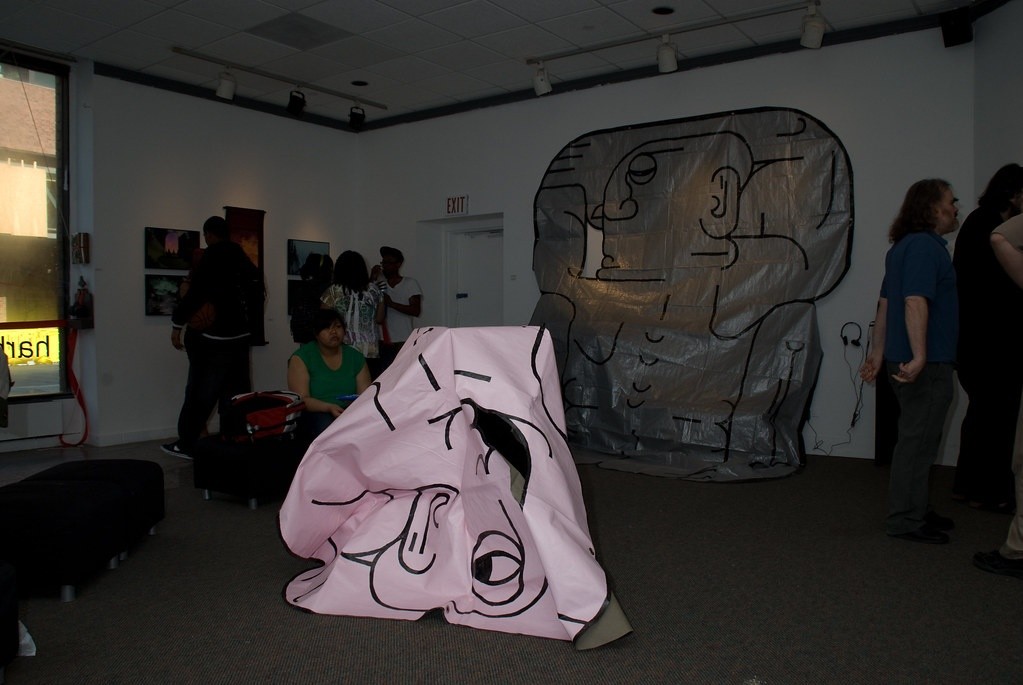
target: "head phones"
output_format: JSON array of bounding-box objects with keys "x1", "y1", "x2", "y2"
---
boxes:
[{"x1": 840, "y1": 321, "x2": 862, "y2": 346}]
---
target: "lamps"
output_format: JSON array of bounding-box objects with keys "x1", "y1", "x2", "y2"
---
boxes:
[
  {"x1": 216, "y1": 71, "x2": 236, "y2": 99},
  {"x1": 347, "y1": 107, "x2": 365, "y2": 133},
  {"x1": 531, "y1": 68, "x2": 552, "y2": 96},
  {"x1": 657, "y1": 35, "x2": 678, "y2": 74},
  {"x1": 799, "y1": 15, "x2": 826, "y2": 49},
  {"x1": 284, "y1": 91, "x2": 306, "y2": 117}
]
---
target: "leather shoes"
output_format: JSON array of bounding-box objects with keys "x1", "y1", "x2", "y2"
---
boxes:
[
  {"x1": 887, "y1": 523, "x2": 949, "y2": 544},
  {"x1": 922, "y1": 510, "x2": 956, "y2": 531}
]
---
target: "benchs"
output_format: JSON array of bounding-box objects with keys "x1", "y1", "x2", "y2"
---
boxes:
[{"x1": 192, "y1": 432, "x2": 316, "y2": 514}]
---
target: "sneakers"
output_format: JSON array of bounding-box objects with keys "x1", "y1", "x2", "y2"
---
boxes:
[{"x1": 160, "y1": 439, "x2": 193, "y2": 460}]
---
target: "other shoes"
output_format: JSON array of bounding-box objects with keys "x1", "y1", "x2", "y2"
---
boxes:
[
  {"x1": 973, "y1": 550, "x2": 1023, "y2": 580},
  {"x1": 953, "y1": 493, "x2": 1016, "y2": 515}
]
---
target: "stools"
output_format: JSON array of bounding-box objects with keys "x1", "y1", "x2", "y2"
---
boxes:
[{"x1": 0, "y1": 460, "x2": 165, "y2": 685}]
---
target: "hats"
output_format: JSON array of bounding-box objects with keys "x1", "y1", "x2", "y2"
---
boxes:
[{"x1": 380, "y1": 246, "x2": 404, "y2": 263}]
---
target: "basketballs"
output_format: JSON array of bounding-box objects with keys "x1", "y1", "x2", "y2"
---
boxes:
[{"x1": 188, "y1": 304, "x2": 216, "y2": 331}]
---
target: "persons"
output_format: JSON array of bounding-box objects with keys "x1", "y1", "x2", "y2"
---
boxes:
[
  {"x1": 290, "y1": 245, "x2": 422, "y2": 380},
  {"x1": 952, "y1": 161, "x2": 1023, "y2": 580},
  {"x1": 0, "y1": 344, "x2": 10, "y2": 428},
  {"x1": 286, "y1": 308, "x2": 372, "y2": 440},
  {"x1": 858, "y1": 178, "x2": 961, "y2": 538},
  {"x1": 159, "y1": 215, "x2": 262, "y2": 457}
]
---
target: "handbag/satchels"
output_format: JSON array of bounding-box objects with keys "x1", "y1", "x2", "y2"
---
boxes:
[{"x1": 218, "y1": 390, "x2": 305, "y2": 445}]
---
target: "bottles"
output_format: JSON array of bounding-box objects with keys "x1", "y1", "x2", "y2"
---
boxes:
[{"x1": 75, "y1": 288, "x2": 90, "y2": 319}]
